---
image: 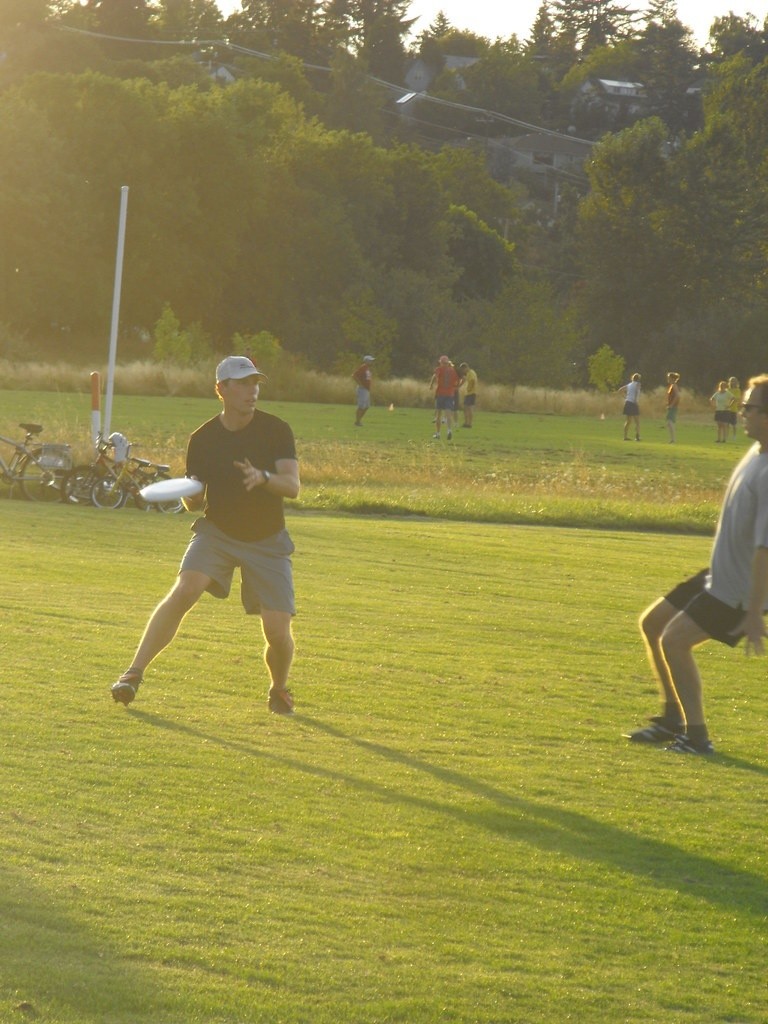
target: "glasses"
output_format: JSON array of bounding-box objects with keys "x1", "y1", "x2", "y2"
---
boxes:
[{"x1": 741, "y1": 401, "x2": 765, "y2": 413}]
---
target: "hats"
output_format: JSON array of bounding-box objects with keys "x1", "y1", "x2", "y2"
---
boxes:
[
  {"x1": 364, "y1": 355, "x2": 376, "y2": 361},
  {"x1": 215, "y1": 356, "x2": 269, "y2": 384}
]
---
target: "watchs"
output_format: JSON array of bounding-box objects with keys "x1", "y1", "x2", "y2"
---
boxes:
[{"x1": 260, "y1": 470, "x2": 271, "y2": 488}]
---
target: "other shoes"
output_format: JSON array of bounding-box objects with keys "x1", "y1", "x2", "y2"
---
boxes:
[
  {"x1": 635, "y1": 437, "x2": 641, "y2": 441},
  {"x1": 669, "y1": 440, "x2": 675, "y2": 444},
  {"x1": 715, "y1": 439, "x2": 726, "y2": 442},
  {"x1": 433, "y1": 434, "x2": 440, "y2": 438},
  {"x1": 355, "y1": 421, "x2": 363, "y2": 426},
  {"x1": 461, "y1": 424, "x2": 472, "y2": 428},
  {"x1": 624, "y1": 437, "x2": 631, "y2": 440},
  {"x1": 447, "y1": 430, "x2": 452, "y2": 440}
]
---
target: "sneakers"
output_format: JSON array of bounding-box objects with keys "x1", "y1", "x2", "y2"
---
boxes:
[
  {"x1": 664, "y1": 735, "x2": 714, "y2": 754},
  {"x1": 622, "y1": 717, "x2": 684, "y2": 742},
  {"x1": 111, "y1": 669, "x2": 142, "y2": 704},
  {"x1": 269, "y1": 687, "x2": 295, "y2": 713}
]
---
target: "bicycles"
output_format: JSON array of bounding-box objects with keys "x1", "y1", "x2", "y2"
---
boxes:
[
  {"x1": 0, "y1": 422, "x2": 75, "y2": 505},
  {"x1": 61, "y1": 430, "x2": 186, "y2": 515}
]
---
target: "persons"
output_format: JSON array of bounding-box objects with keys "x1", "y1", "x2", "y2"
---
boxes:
[
  {"x1": 710, "y1": 377, "x2": 741, "y2": 443},
  {"x1": 618, "y1": 373, "x2": 642, "y2": 441},
  {"x1": 429, "y1": 355, "x2": 478, "y2": 440},
  {"x1": 664, "y1": 373, "x2": 680, "y2": 444},
  {"x1": 112, "y1": 356, "x2": 301, "y2": 713},
  {"x1": 351, "y1": 356, "x2": 377, "y2": 427},
  {"x1": 628, "y1": 372, "x2": 768, "y2": 756}
]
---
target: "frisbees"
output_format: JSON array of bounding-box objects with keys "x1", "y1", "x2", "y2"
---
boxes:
[{"x1": 139, "y1": 478, "x2": 204, "y2": 499}]
